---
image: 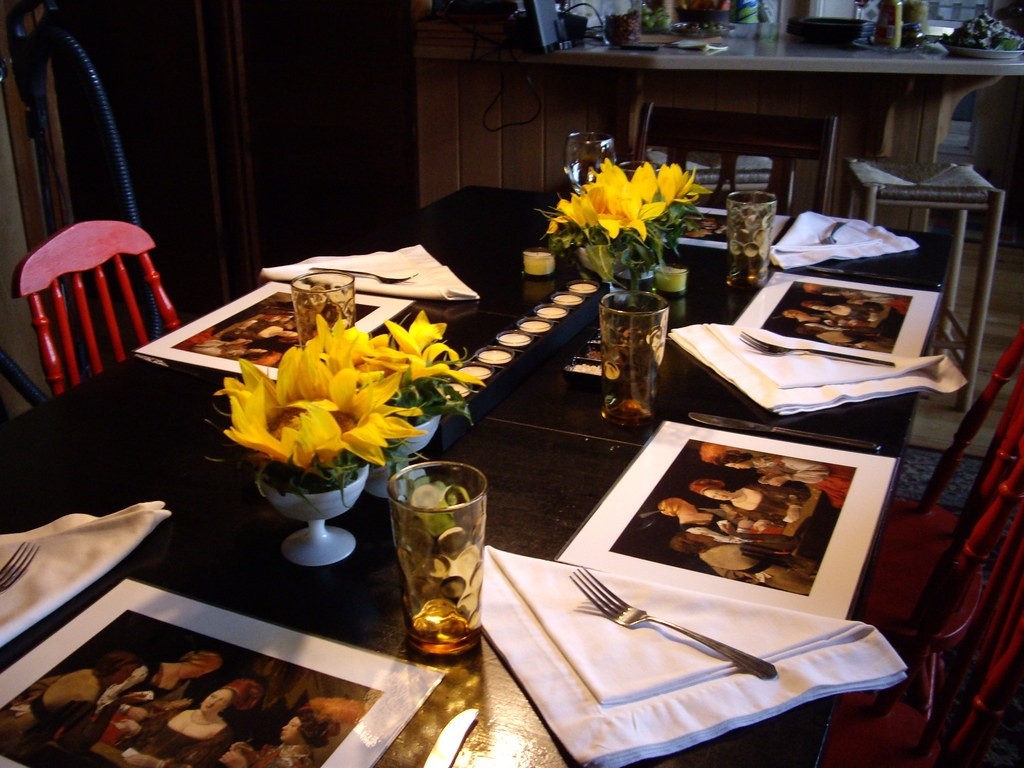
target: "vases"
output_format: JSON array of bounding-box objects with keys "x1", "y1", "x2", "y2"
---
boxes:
[
  {"x1": 368, "y1": 404, "x2": 446, "y2": 507},
  {"x1": 677, "y1": 9, "x2": 730, "y2": 24},
  {"x1": 255, "y1": 456, "x2": 375, "y2": 568}
]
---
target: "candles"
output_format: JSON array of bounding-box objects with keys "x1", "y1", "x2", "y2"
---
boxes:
[
  {"x1": 654, "y1": 262, "x2": 689, "y2": 298},
  {"x1": 523, "y1": 246, "x2": 555, "y2": 282}
]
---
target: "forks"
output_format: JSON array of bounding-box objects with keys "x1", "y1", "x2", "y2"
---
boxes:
[
  {"x1": 0, "y1": 542, "x2": 40, "y2": 596},
  {"x1": 738, "y1": 331, "x2": 895, "y2": 367},
  {"x1": 816, "y1": 221, "x2": 846, "y2": 245},
  {"x1": 309, "y1": 267, "x2": 419, "y2": 284},
  {"x1": 569, "y1": 566, "x2": 777, "y2": 682}
]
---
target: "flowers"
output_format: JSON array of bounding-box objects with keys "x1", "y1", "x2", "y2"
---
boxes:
[
  {"x1": 530, "y1": 156, "x2": 713, "y2": 287},
  {"x1": 206, "y1": 306, "x2": 477, "y2": 511}
]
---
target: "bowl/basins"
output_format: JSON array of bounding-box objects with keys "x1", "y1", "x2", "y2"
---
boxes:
[{"x1": 675, "y1": 6, "x2": 730, "y2": 23}]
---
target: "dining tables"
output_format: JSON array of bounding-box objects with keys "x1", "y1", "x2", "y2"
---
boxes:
[{"x1": 0, "y1": 178, "x2": 965, "y2": 768}]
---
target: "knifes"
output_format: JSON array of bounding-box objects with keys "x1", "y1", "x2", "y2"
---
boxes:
[{"x1": 688, "y1": 412, "x2": 881, "y2": 450}]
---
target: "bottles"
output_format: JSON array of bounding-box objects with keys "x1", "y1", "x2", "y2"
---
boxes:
[{"x1": 902, "y1": 21, "x2": 924, "y2": 48}]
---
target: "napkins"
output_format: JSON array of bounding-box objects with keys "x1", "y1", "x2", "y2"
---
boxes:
[
  {"x1": 480, "y1": 542, "x2": 910, "y2": 768},
  {"x1": 0, "y1": 495, "x2": 173, "y2": 649},
  {"x1": 667, "y1": 313, "x2": 970, "y2": 416},
  {"x1": 768, "y1": 208, "x2": 924, "y2": 271},
  {"x1": 250, "y1": 239, "x2": 480, "y2": 310}
]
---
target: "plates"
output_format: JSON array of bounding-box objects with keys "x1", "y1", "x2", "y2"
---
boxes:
[
  {"x1": 673, "y1": 23, "x2": 735, "y2": 39},
  {"x1": 940, "y1": 43, "x2": 1024, "y2": 60},
  {"x1": 786, "y1": 16, "x2": 874, "y2": 45}
]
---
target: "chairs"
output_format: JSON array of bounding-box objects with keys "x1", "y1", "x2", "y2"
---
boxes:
[
  {"x1": 817, "y1": 456, "x2": 1024, "y2": 768},
  {"x1": 853, "y1": 316, "x2": 1024, "y2": 706},
  {"x1": 7, "y1": 213, "x2": 181, "y2": 390},
  {"x1": 628, "y1": 99, "x2": 843, "y2": 216}
]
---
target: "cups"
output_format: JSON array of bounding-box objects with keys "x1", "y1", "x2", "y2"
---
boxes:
[
  {"x1": 291, "y1": 271, "x2": 356, "y2": 349},
  {"x1": 726, "y1": 191, "x2": 777, "y2": 288},
  {"x1": 564, "y1": 131, "x2": 616, "y2": 195},
  {"x1": 602, "y1": 0, "x2": 642, "y2": 44},
  {"x1": 618, "y1": 160, "x2": 661, "y2": 183},
  {"x1": 599, "y1": 289, "x2": 669, "y2": 426},
  {"x1": 385, "y1": 461, "x2": 489, "y2": 655}
]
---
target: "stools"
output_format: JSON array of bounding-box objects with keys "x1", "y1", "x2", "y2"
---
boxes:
[{"x1": 837, "y1": 151, "x2": 1007, "y2": 416}]
